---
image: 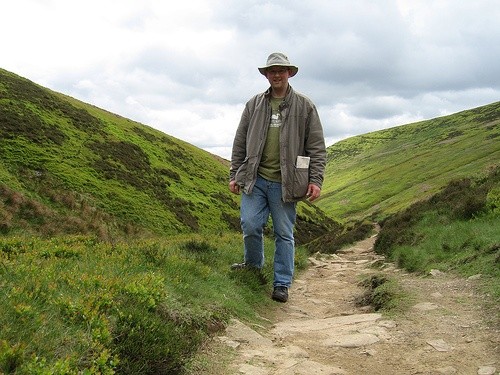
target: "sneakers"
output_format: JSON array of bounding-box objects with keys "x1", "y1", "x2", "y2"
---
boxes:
[
  {"x1": 272, "y1": 286, "x2": 288, "y2": 302},
  {"x1": 232, "y1": 263, "x2": 247, "y2": 269}
]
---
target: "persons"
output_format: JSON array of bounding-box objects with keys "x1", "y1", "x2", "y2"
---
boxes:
[{"x1": 228, "y1": 52, "x2": 327, "y2": 303}]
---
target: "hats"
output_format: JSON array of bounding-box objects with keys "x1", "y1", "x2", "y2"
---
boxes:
[{"x1": 258, "y1": 53, "x2": 298, "y2": 78}]
